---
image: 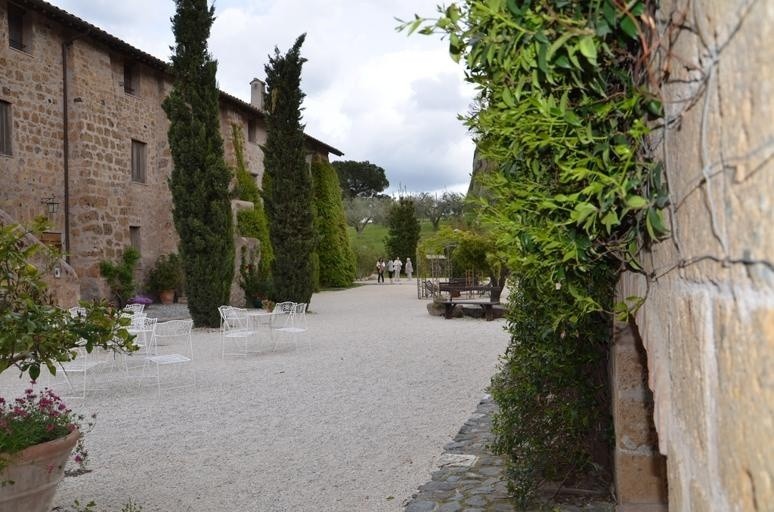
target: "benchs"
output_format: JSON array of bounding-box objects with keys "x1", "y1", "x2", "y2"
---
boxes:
[{"x1": 434, "y1": 285, "x2": 501, "y2": 321}]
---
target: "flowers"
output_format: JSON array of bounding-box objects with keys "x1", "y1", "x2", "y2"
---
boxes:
[{"x1": 1, "y1": 378, "x2": 91, "y2": 486}]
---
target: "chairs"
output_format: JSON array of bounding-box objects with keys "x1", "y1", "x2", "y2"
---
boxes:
[{"x1": 44, "y1": 302, "x2": 309, "y2": 402}]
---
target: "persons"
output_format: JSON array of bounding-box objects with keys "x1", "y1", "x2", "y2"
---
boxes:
[
  {"x1": 374, "y1": 256, "x2": 386, "y2": 285},
  {"x1": 392, "y1": 255, "x2": 402, "y2": 281},
  {"x1": 385, "y1": 258, "x2": 394, "y2": 284},
  {"x1": 403, "y1": 257, "x2": 413, "y2": 280}
]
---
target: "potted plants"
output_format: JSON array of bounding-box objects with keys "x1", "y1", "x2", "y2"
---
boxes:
[
  {"x1": 150, "y1": 252, "x2": 183, "y2": 304},
  {"x1": 238, "y1": 264, "x2": 272, "y2": 309}
]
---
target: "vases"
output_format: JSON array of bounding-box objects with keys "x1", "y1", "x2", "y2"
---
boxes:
[{"x1": 0, "y1": 428, "x2": 82, "y2": 512}]
